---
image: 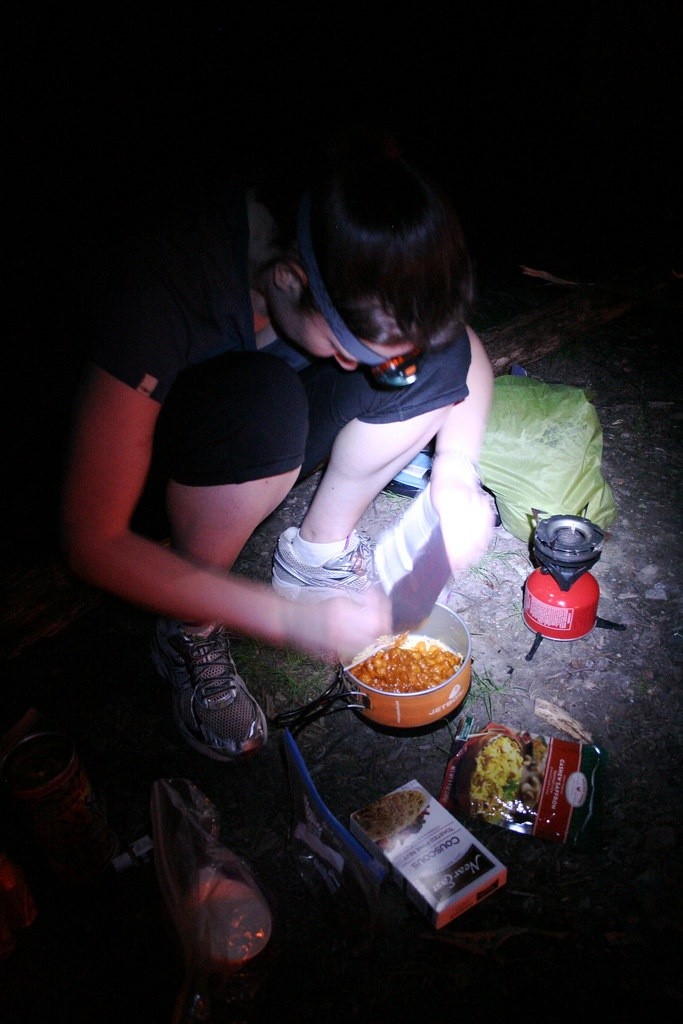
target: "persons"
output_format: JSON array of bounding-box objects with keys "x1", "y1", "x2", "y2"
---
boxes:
[{"x1": 57, "y1": 139, "x2": 500, "y2": 767}]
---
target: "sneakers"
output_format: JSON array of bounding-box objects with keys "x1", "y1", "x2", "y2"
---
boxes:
[
  {"x1": 270, "y1": 525, "x2": 449, "y2": 606},
  {"x1": 150, "y1": 614, "x2": 268, "y2": 760}
]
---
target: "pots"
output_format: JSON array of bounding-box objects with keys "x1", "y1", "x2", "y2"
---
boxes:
[{"x1": 273, "y1": 601, "x2": 471, "y2": 739}]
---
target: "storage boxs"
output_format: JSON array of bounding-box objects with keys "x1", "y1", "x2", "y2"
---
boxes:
[{"x1": 348, "y1": 780, "x2": 507, "y2": 930}]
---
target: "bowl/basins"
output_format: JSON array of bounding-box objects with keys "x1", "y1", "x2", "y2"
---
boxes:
[{"x1": 453, "y1": 732, "x2": 525, "y2": 819}]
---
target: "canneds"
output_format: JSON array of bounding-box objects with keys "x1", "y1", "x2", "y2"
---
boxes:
[{"x1": 0, "y1": 731, "x2": 122, "y2": 886}]
